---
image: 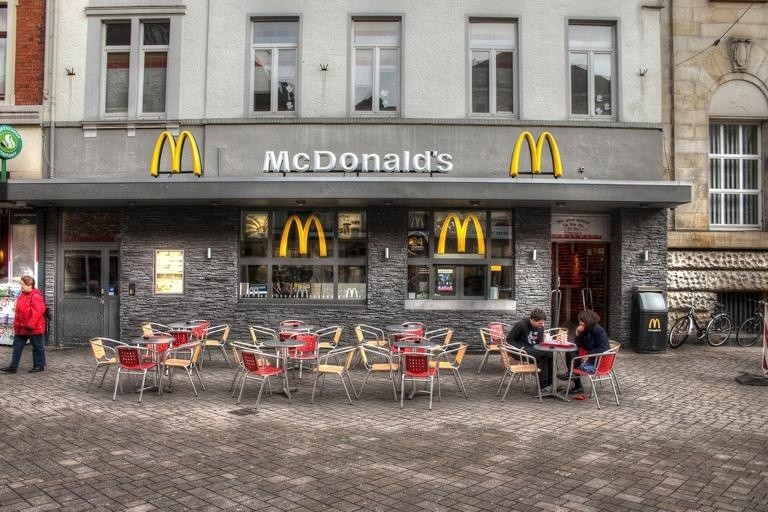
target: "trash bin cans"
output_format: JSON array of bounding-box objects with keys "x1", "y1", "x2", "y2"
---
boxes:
[{"x1": 631, "y1": 285, "x2": 668, "y2": 354}]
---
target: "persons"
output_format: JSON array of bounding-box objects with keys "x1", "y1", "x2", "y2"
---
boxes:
[
  {"x1": 1, "y1": 275, "x2": 47, "y2": 373},
  {"x1": 555, "y1": 309, "x2": 609, "y2": 394},
  {"x1": 507, "y1": 308, "x2": 553, "y2": 393}
]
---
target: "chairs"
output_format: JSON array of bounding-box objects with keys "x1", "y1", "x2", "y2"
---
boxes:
[
  {"x1": 135, "y1": 333, "x2": 173, "y2": 388},
  {"x1": 198, "y1": 324, "x2": 233, "y2": 371},
  {"x1": 496, "y1": 345, "x2": 543, "y2": 403},
  {"x1": 285, "y1": 333, "x2": 319, "y2": 379},
  {"x1": 544, "y1": 327, "x2": 568, "y2": 342},
  {"x1": 398, "y1": 337, "x2": 432, "y2": 364},
  {"x1": 357, "y1": 343, "x2": 399, "y2": 402},
  {"x1": 424, "y1": 341, "x2": 469, "y2": 402},
  {"x1": 426, "y1": 328, "x2": 453, "y2": 361},
  {"x1": 229, "y1": 341, "x2": 270, "y2": 391},
  {"x1": 397, "y1": 352, "x2": 435, "y2": 411},
  {"x1": 141, "y1": 322, "x2": 174, "y2": 369},
  {"x1": 402, "y1": 322, "x2": 424, "y2": 339},
  {"x1": 277, "y1": 332, "x2": 303, "y2": 379},
  {"x1": 488, "y1": 322, "x2": 512, "y2": 343},
  {"x1": 237, "y1": 351, "x2": 291, "y2": 407},
  {"x1": 501, "y1": 342, "x2": 534, "y2": 393},
  {"x1": 592, "y1": 340, "x2": 621, "y2": 394},
  {"x1": 186, "y1": 320, "x2": 212, "y2": 361},
  {"x1": 86, "y1": 337, "x2": 129, "y2": 394},
  {"x1": 158, "y1": 340, "x2": 206, "y2": 396},
  {"x1": 311, "y1": 325, "x2": 343, "y2": 373},
  {"x1": 112, "y1": 345, "x2": 163, "y2": 402},
  {"x1": 280, "y1": 320, "x2": 308, "y2": 368},
  {"x1": 165, "y1": 329, "x2": 194, "y2": 376},
  {"x1": 476, "y1": 328, "x2": 512, "y2": 374},
  {"x1": 353, "y1": 324, "x2": 389, "y2": 369},
  {"x1": 388, "y1": 333, "x2": 418, "y2": 377},
  {"x1": 564, "y1": 352, "x2": 619, "y2": 409},
  {"x1": 310, "y1": 345, "x2": 357, "y2": 405},
  {"x1": 248, "y1": 324, "x2": 279, "y2": 376}
]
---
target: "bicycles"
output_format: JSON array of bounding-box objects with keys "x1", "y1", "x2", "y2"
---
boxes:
[
  {"x1": 669, "y1": 287, "x2": 733, "y2": 346},
  {"x1": 736, "y1": 298, "x2": 768, "y2": 347}
]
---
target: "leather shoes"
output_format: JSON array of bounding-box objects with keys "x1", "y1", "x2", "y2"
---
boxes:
[
  {"x1": 0, "y1": 366, "x2": 17, "y2": 374},
  {"x1": 563, "y1": 386, "x2": 583, "y2": 395},
  {"x1": 556, "y1": 373, "x2": 575, "y2": 381},
  {"x1": 28, "y1": 365, "x2": 44, "y2": 373}
]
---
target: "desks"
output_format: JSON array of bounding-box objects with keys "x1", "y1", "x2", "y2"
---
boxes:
[
  {"x1": 533, "y1": 341, "x2": 578, "y2": 401},
  {"x1": 393, "y1": 341, "x2": 436, "y2": 400},
  {"x1": 167, "y1": 322, "x2": 202, "y2": 330},
  {"x1": 278, "y1": 324, "x2": 315, "y2": 371},
  {"x1": 263, "y1": 340, "x2": 305, "y2": 398},
  {"x1": 385, "y1": 325, "x2": 420, "y2": 334},
  {"x1": 132, "y1": 336, "x2": 175, "y2": 393}
]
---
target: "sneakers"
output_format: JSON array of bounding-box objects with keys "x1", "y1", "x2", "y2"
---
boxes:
[
  {"x1": 550, "y1": 383, "x2": 564, "y2": 392},
  {"x1": 540, "y1": 386, "x2": 554, "y2": 394}
]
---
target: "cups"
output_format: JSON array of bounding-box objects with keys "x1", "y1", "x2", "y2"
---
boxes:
[
  {"x1": 543, "y1": 333, "x2": 551, "y2": 343},
  {"x1": 560, "y1": 332, "x2": 567, "y2": 344}
]
